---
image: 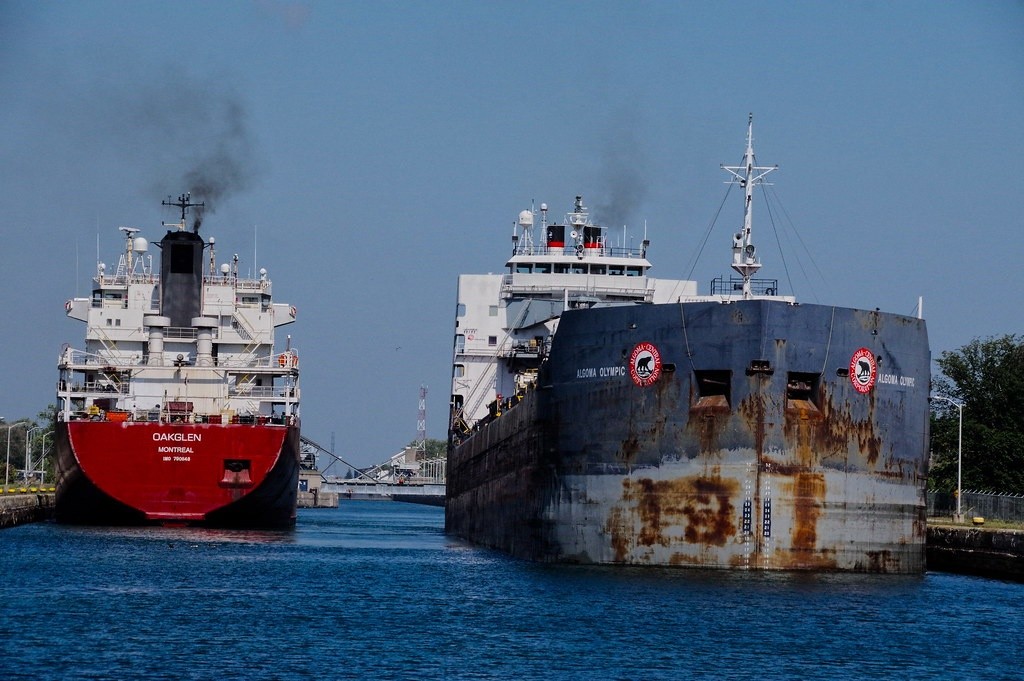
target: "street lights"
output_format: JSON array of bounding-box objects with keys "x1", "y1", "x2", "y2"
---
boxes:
[
  {"x1": 933, "y1": 396, "x2": 964, "y2": 523},
  {"x1": 6, "y1": 422, "x2": 54, "y2": 485}
]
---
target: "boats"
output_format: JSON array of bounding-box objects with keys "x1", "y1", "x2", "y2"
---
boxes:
[
  {"x1": 56, "y1": 192, "x2": 301, "y2": 528},
  {"x1": 446, "y1": 111, "x2": 931, "y2": 573}
]
